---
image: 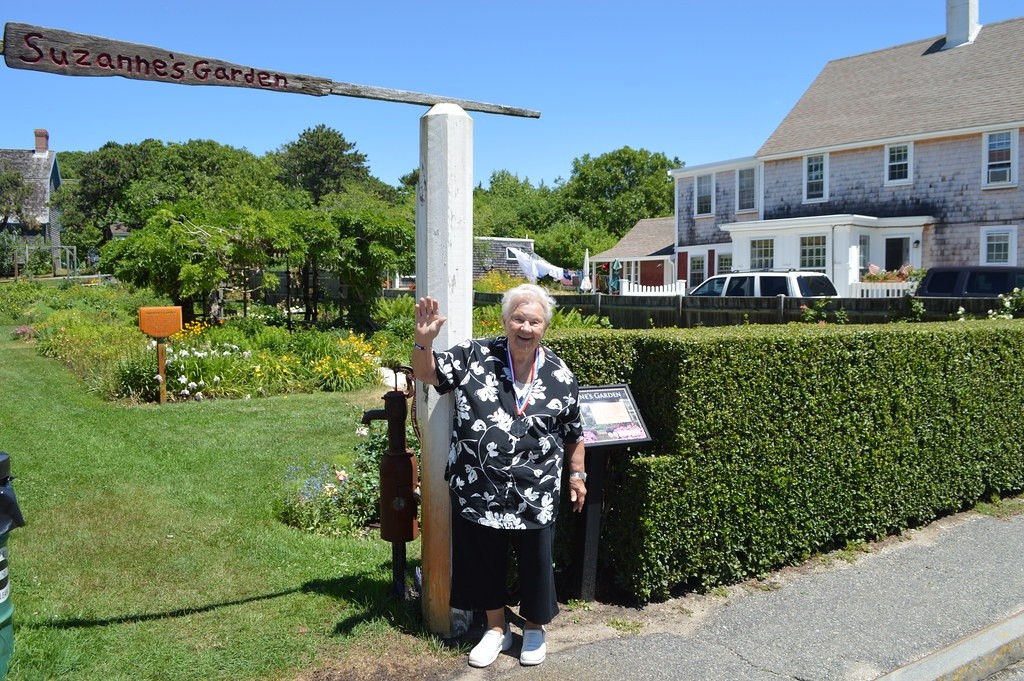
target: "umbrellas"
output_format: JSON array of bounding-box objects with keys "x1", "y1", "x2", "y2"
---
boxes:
[
  {"x1": 610, "y1": 258, "x2": 623, "y2": 291},
  {"x1": 580, "y1": 248, "x2": 593, "y2": 291}
]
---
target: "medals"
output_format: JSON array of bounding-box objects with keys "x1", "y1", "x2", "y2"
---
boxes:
[{"x1": 510, "y1": 418, "x2": 528, "y2": 439}]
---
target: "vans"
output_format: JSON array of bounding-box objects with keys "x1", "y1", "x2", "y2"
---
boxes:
[{"x1": 915, "y1": 266, "x2": 1024, "y2": 297}]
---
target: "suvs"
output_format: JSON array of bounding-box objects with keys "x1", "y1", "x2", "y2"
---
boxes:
[{"x1": 687, "y1": 268, "x2": 840, "y2": 297}]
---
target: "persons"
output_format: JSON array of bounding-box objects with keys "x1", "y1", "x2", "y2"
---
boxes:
[{"x1": 413, "y1": 283, "x2": 588, "y2": 669}]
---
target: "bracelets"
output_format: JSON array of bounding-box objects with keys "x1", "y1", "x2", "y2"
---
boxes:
[
  {"x1": 568, "y1": 470, "x2": 588, "y2": 482},
  {"x1": 415, "y1": 341, "x2": 434, "y2": 351}
]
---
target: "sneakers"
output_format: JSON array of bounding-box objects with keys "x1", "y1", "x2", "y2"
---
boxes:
[
  {"x1": 519, "y1": 624, "x2": 547, "y2": 666},
  {"x1": 469, "y1": 624, "x2": 513, "y2": 668}
]
